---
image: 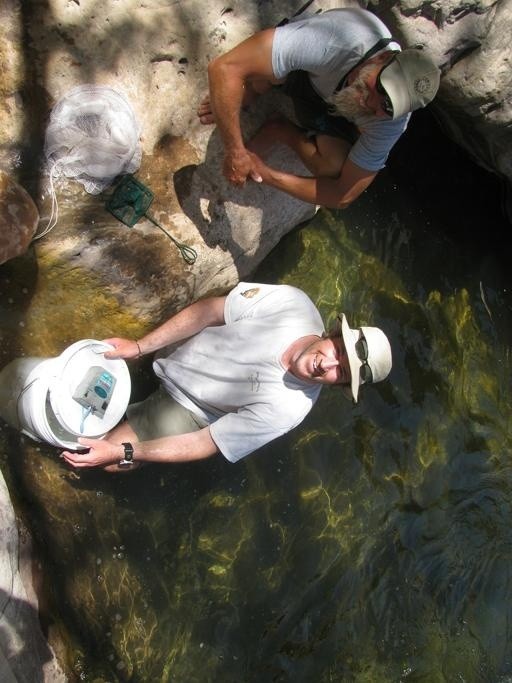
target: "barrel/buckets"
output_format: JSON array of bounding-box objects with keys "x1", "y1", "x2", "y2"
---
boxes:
[{"x1": 0, "y1": 338, "x2": 133, "y2": 452}]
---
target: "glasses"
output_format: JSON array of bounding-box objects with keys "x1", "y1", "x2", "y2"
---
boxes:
[
  {"x1": 354, "y1": 328, "x2": 374, "y2": 384},
  {"x1": 374, "y1": 62, "x2": 393, "y2": 117}
]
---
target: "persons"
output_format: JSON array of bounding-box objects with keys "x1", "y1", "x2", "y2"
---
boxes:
[
  {"x1": 58, "y1": 279, "x2": 393, "y2": 474},
  {"x1": 198, "y1": 4, "x2": 443, "y2": 213}
]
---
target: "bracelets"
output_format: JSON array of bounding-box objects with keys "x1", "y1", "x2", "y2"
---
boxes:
[
  {"x1": 118, "y1": 439, "x2": 135, "y2": 466},
  {"x1": 135, "y1": 339, "x2": 143, "y2": 358}
]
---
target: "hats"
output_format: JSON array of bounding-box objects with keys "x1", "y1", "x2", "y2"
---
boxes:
[
  {"x1": 380, "y1": 48, "x2": 441, "y2": 120},
  {"x1": 337, "y1": 313, "x2": 393, "y2": 404}
]
